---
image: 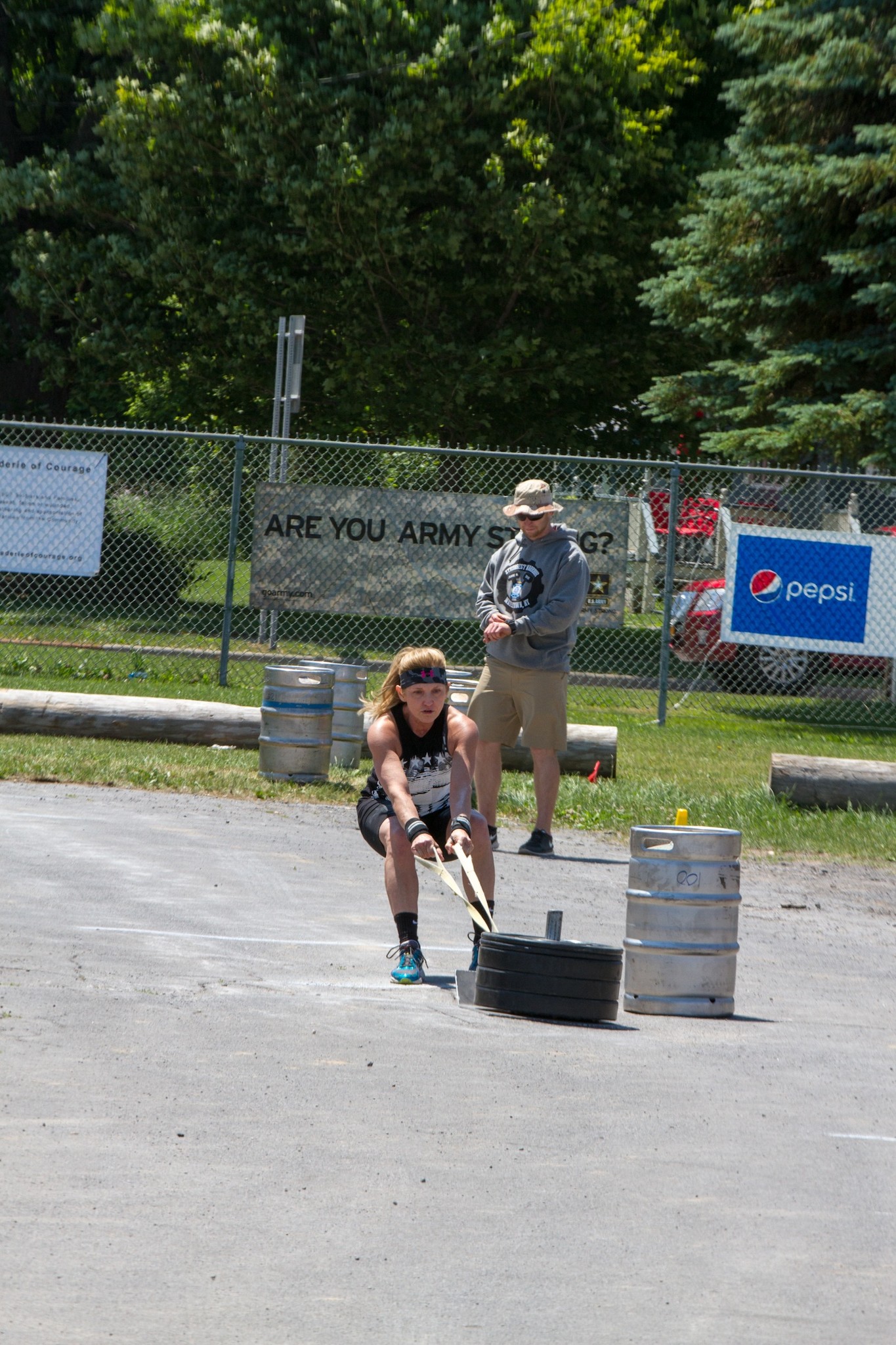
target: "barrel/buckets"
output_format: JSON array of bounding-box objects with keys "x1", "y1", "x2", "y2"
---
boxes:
[
  {"x1": 257, "y1": 665, "x2": 335, "y2": 786},
  {"x1": 623, "y1": 825, "x2": 742, "y2": 1018},
  {"x1": 302, "y1": 659, "x2": 370, "y2": 770},
  {"x1": 445, "y1": 669, "x2": 474, "y2": 717}
]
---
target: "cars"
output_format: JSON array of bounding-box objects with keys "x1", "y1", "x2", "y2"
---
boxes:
[{"x1": 668, "y1": 524, "x2": 896, "y2": 693}]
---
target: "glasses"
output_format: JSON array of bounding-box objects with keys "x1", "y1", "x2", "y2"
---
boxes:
[{"x1": 516, "y1": 513, "x2": 545, "y2": 521}]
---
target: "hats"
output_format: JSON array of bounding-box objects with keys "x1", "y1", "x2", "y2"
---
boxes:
[{"x1": 502, "y1": 479, "x2": 565, "y2": 516}]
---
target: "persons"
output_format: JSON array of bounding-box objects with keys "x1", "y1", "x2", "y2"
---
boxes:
[
  {"x1": 465, "y1": 479, "x2": 591, "y2": 856},
  {"x1": 356, "y1": 645, "x2": 497, "y2": 985}
]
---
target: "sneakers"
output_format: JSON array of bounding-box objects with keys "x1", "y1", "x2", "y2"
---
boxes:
[
  {"x1": 468, "y1": 931, "x2": 481, "y2": 971},
  {"x1": 386, "y1": 940, "x2": 428, "y2": 984},
  {"x1": 487, "y1": 825, "x2": 499, "y2": 849},
  {"x1": 518, "y1": 828, "x2": 554, "y2": 855}
]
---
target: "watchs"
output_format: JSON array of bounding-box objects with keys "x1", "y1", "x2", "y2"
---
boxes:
[{"x1": 505, "y1": 619, "x2": 516, "y2": 634}]
